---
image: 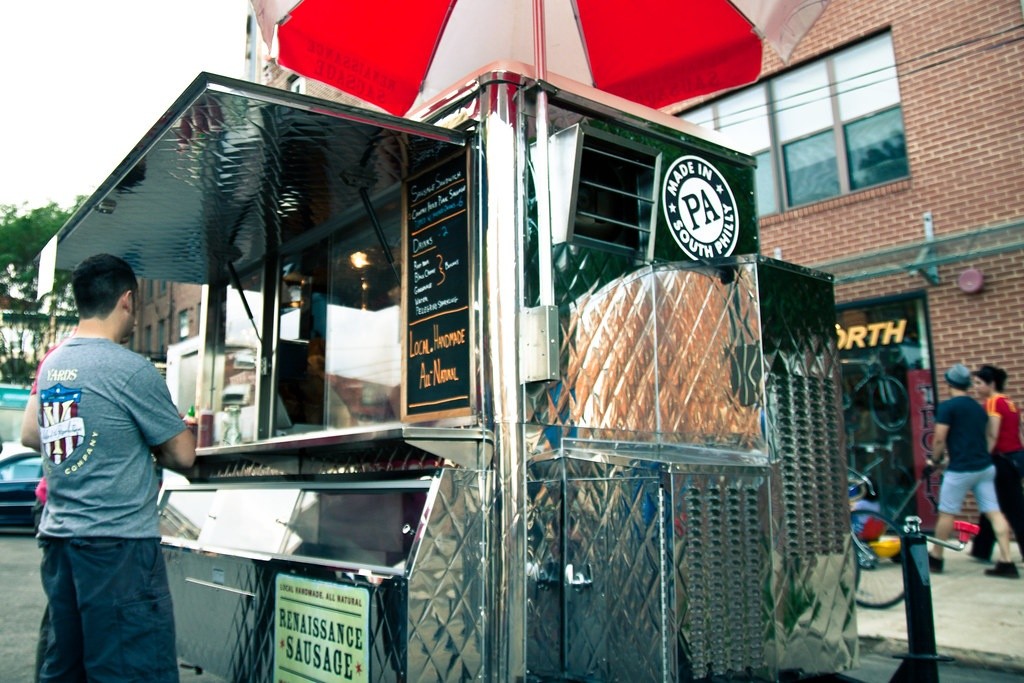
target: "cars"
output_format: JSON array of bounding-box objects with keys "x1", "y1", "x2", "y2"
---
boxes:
[{"x1": 0, "y1": 452, "x2": 45, "y2": 528}]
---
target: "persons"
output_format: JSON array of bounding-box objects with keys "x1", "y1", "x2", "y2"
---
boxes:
[
  {"x1": 926, "y1": 365, "x2": 1020, "y2": 579},
  {"x1": 306, "y1": 337, "x2": 400, "y2": 427},
  {"x1": 965, "y1": 365, "x2": 1024, "y2": 563},
  {"x1": 18, "y1": 253, "x2": 195, "y2": 683}
]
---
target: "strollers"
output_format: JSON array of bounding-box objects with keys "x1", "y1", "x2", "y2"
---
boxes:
[{"x1": 849, "y1": 453, "x2": 941, "y2": 570}]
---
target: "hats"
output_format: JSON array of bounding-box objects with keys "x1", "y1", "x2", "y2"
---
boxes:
[
  {"x1": 944, "y1": 364, "x2": 971, "y2": 390},
  {"x1": 969, "y1": 365, "x2": 1006, "y2": 386}
]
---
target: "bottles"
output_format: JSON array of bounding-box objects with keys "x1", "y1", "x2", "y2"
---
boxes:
[
  {"x1": 221, "y1": 404, "x2": 242, "y2": 445},
  {"x1": 182, "y1": 404, "x2": 198, "y2": 449},
  {"x1": 198, "y1": 408, "x2": 213, "y2": 449}
]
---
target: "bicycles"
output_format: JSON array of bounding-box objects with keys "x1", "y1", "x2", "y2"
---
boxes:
[{"x1": 846, "y1": 464, "x2": 905, "y2": 611}]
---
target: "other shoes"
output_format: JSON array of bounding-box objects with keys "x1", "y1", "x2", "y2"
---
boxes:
[
  {"x1": 928, "y1": 553, "x2": 944, "y2": 573},
  {"x1": 1015, "y1": 561, "x2": 1024, "y2": 567},
  {"x1": 986, "y1": 561, "x2": 1020, "y2": 579}
]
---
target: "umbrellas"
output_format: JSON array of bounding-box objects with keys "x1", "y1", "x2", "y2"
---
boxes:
[{"x1": 248, "y1": 1, "x2": 833, "y2": 306}]
